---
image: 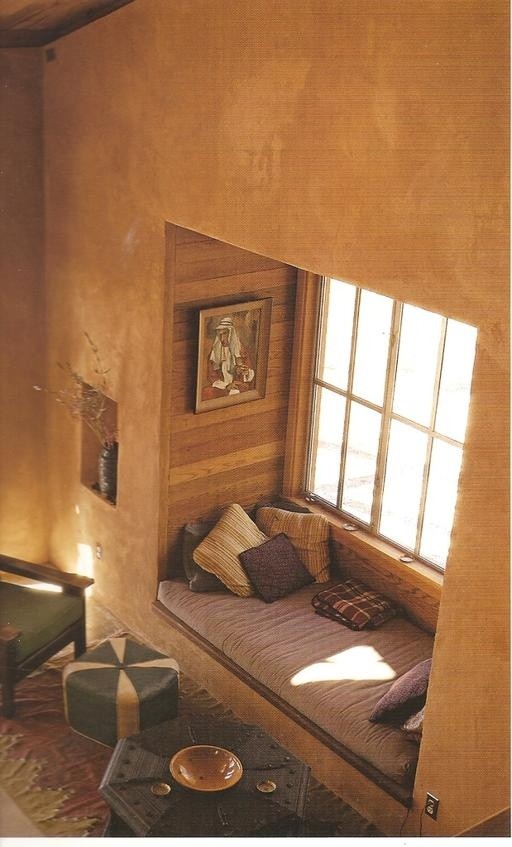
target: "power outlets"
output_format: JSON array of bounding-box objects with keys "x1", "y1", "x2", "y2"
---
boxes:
[{"x1": 424, "y1": 791, "x2": 440, "y2": 821}]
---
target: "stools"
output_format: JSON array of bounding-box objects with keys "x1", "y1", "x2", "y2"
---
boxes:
[{"x1": 63, "y1": 635, "x2": 182, "y2": 752}]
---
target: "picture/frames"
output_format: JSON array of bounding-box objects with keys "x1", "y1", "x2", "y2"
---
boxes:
[{"x1": 190, "y1": 297, "x2": 275, "y2": 413}]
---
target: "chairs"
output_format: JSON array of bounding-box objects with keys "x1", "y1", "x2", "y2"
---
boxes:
[{"x1": 0, "y1": 552, "x2": 95, "y2": 720}]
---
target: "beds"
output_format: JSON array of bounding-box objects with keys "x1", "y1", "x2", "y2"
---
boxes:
[{"x1": 157, "y1": 548, "x2": 436, "y2": 837}]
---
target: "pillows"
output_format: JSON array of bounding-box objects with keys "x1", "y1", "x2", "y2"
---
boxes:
[
  {"x1": 182, "y1": 494, "x2": 329, "y2": 604},
  {"x1": 370, "y1": 655, "x2": 429, "y2": 736}
]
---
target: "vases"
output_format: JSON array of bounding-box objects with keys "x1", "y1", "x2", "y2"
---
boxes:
[{"x1": 99, "y1": 442, "x2": 121, "y2": 503}]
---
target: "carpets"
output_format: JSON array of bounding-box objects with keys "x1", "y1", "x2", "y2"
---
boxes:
[{"x1": 1, "y1": 627, "x2": 384, "y2": 838}]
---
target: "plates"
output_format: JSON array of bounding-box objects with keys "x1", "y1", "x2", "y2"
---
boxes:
[
  {"x1": 256, "y1": 780, "x2": 276, "y2": 793},
  {"x1": 150, "y1": 783, "x2": 170, "y2": 797},
  {"x1": 399, "y1": 554, "x2": 415, "y2": 563},
  {"x1": 342, "y1": 523, "x2": 359, "y2": 532}
]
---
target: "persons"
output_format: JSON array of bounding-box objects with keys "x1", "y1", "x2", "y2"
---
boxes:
[{"x1": 201, "y1": 317, "x2": 256, "y2": 401}]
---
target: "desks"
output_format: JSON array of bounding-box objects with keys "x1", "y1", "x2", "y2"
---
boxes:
[{"x1": 98, "y1": 714, "x2": 310, "y2": 836}]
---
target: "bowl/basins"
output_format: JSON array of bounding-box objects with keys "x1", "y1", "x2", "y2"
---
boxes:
[{"x1": 169, "y1": 745, "x2": 244, "y2": 793}]
